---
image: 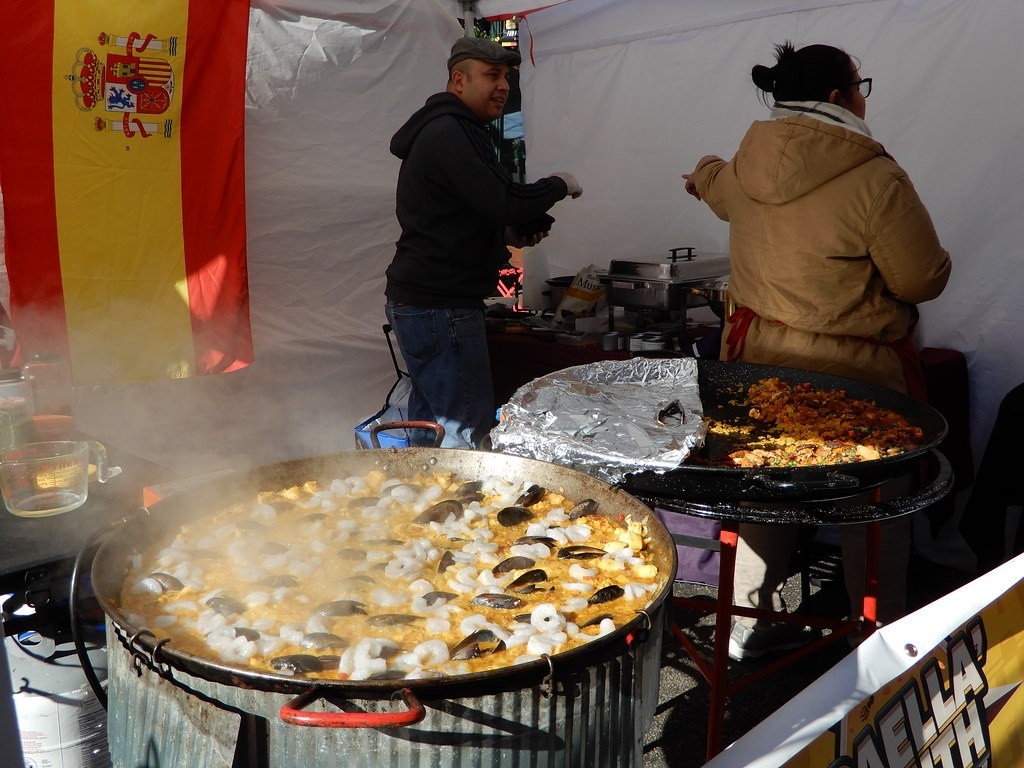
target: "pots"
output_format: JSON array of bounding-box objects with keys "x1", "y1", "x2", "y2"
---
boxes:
[
  {"x1": 514, "y1": 358, "x2": 948, "y2": 507},
  {"x1": 90, "y1": 420, "x2": 679, "y2": 729}
]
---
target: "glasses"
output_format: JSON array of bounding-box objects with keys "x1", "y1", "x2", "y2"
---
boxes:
[{"x1": 853, "y1": 78, "x2": 872, "y2": 98}]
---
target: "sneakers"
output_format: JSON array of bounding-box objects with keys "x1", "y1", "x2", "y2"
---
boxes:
[{"x1": 721, "y1": 617, "x2": 822, "y2": 658}]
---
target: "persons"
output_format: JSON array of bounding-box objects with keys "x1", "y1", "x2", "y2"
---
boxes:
[
  {"x1": 385, "y1": 36, "x2": 583, "y2": 451},
  {"x1": 681, "y1": 43, "x2": 952, "y2": 663}
]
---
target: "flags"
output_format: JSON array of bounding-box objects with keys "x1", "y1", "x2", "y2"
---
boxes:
[{"x1": 0, "y1": 0, "x2": 255, "y2": 382}]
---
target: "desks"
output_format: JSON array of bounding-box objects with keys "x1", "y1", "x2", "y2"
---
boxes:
[
  {"x1": 486, "y1": 315, "x2": 723, "y2": 432},
  {"x1": 0, "y1": 442, "x2": 191, "y2": 768}
]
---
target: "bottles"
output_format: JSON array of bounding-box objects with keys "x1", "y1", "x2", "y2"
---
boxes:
[
  {"x1": 0, "y1": 367, "x2": 34, "y2": 451},
  {"x1": 19, "y1": 351, "x2": 77, "y2": 443}
]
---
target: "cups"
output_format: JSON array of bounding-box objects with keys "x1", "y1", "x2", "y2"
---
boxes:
[{"x1": 0, "y1": 440, "x2": 109, "y2": 518}]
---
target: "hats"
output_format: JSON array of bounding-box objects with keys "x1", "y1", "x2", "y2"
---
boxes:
[{"x1": 447, "y1": 37, "x2": 522, "y2": 71}]
